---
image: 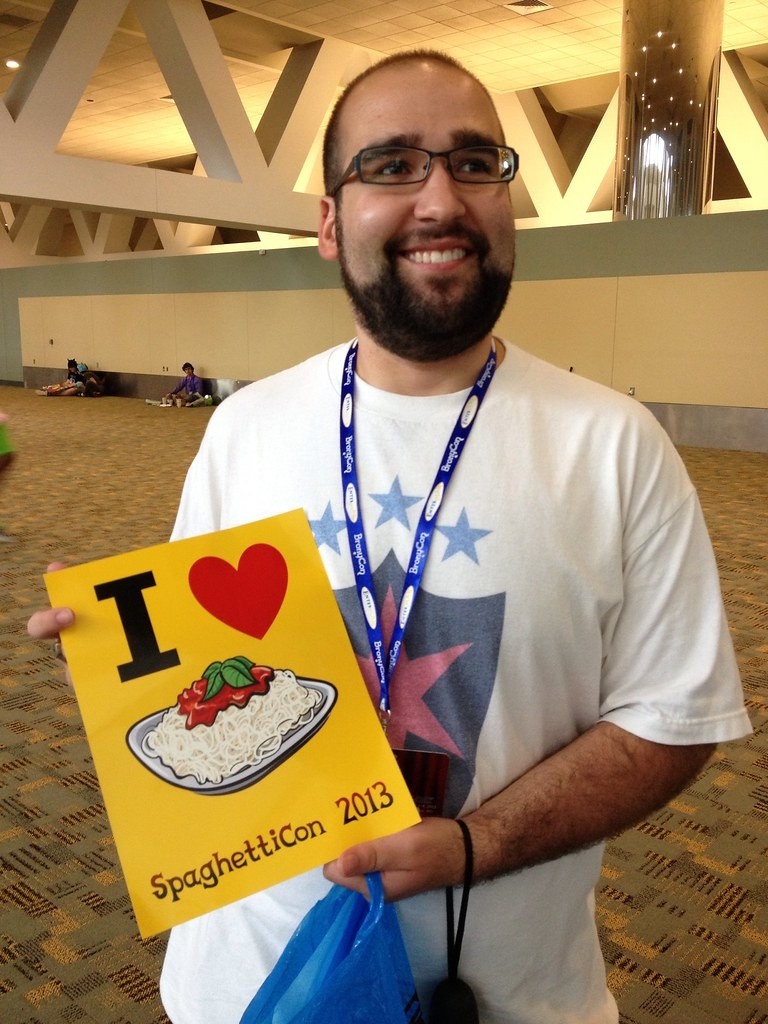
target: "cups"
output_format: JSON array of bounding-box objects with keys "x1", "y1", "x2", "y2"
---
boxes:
[
  {"x1": 169, "y1": 400, "x2": 173, "y2": 405},
  {"x1": 177, "y1": 399, "x2": 181, "y2": 408},
  {"x1": 162, "y1": 397, "x2": 166, "y2": 404}
]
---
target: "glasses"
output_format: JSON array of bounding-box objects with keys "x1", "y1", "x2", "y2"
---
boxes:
[{"x1": 331, "y1": 145, "x2": 520, "y2": 194}]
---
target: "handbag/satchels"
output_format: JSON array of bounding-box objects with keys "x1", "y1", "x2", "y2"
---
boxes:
[{"x1": 237, "y1": 866, "x2": 421, "y2": 1024}]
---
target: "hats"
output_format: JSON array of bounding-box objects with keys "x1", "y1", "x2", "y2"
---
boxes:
[
  {"x1": 77, "y1": 362, "x2": 87, "y2": 372},
  {"x1": 67, "y1": 358, "x2": 77, "y2": 367}
]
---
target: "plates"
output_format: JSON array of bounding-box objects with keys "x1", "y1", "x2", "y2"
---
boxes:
[{"x1": 128, "y1": 680, "x2": 337, "y2": 795}]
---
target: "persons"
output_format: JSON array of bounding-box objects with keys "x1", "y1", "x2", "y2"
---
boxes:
[
  {"x1": 0, "y1": 410, "x2": 19, "y2": 543},
  {"x1": 27, "y1": 52, "x2": 753, "y2": 1024}
]
